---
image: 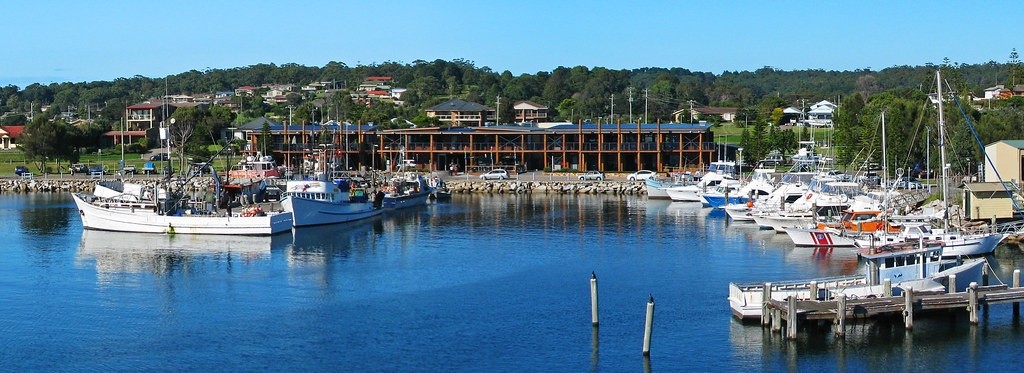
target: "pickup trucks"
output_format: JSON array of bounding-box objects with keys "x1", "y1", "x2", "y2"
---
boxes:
[
  {"x1": 858, "y1": 171, "x2": 881, "y2": 185},
  {"x1": 124, "y1": 164, "x2": 138, "y2": 174},
  {"x1": 142, "y1": 162, "x2": 157, "y2": 175}
]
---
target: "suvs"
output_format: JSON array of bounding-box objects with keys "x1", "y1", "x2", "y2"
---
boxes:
[
  {"x1": 759, "y1": 155, "x2": 786, "y2": 166},
  {"x1": 397, "y1": 159, "x2": 416, "y2": 169},
  {"x1": 15, "y1": 166, "x2": 28, "y2": 176},
  {"x1": 479, "y1": 169, "x2": 510, "y2": 180}
]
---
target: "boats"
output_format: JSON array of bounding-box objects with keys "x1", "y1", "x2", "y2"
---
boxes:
[
  {"x1": 727, "y1": 234, "x2": 987, "y2": 320},
  {"x1": 645, "y1": 70, "x2": 1012, "y2": 258},
  {"x1": 71, "y1": 74, "x2": 451, "y2": 235}
]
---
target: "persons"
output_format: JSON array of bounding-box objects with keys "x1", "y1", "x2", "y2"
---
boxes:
[
  {"x1": 449, "y1": 162, "x2": 458, "y2": 175},
  {"x1": 914, "y1": 164, "x2": 920, "y2": 173}
]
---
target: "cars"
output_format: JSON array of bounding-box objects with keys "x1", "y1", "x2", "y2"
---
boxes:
[
  {"x1": 149, "y1": 152, "x2": 171, "y2": 160},
  {"x1": 71, "y1": 163, "x2": 105, "y2": 175},
  {"x1": 578, "y1": 171, "x2": 605, "y2": 181},
  {"x1": 896, "y1": 177, "x2": 921, "y2": 189},
  {"x1": 825, "y1": 170, "x2": 850, "y2": 182},
  {"x1": 627, "y1": 170, "x2": 657, "y2": 181},
  {"x1": 162, "y1": 164, "x2": 175, "y2": 174}
]
